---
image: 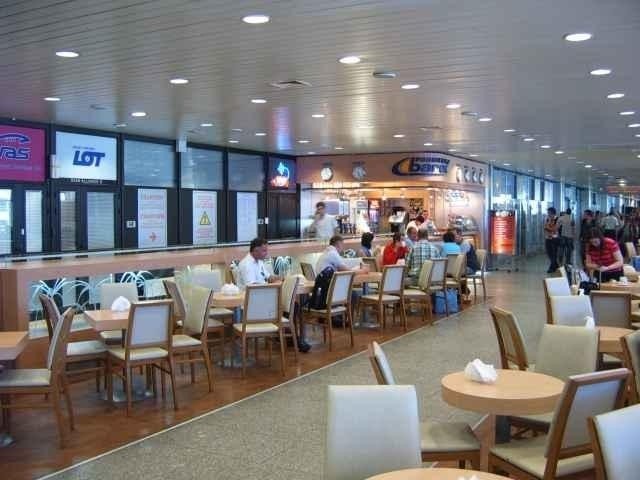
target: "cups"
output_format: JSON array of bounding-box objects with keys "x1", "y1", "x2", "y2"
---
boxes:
[
  {"x1": 620, "y1": 276, "x2": 628, "y2": 286},
  {"x1": 609, "y1": 279, "x2": 616, "y2": 286}
]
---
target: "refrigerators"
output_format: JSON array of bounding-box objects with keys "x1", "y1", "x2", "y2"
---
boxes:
[{"x1": 348, "y1": 199, "x2": 380, "y2": 235}]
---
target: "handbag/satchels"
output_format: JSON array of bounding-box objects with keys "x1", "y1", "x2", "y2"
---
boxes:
[{"x1": 432, "y1": 288, "x2": 459, "y2": 315}]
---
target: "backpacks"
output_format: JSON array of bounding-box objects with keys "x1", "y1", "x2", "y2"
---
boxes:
[{"x1": 309, "y1": 266, "x2": 335, "y2": 310}]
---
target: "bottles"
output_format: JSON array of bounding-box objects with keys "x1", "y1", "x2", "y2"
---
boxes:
[{"x1": 314, "y1": 287, "x2": 322, "y2": 308}]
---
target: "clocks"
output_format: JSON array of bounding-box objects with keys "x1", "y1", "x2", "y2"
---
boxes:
[
  {"x1": 352, "y1": 167, "x2": 367, "y2": 179},
  {"x1": 320, "y1": 168, "x2": 333, "y2": 181}
]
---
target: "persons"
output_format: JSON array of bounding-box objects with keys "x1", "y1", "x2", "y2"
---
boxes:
[
  {"x1": 406, "y1": 216, "x2": 425, "y2": 232},
  {"x1": 419, "y1": 210, "x2": 440, "y2": 235},
  {"x1": 543, "y1": 206, "x2": 625, "y2": 283},
  {"x1": 314, "y1": 226, "x2": 482, "y2": 328},
  {"x1": 235, "y1": 237, "x2": 312, "y2": 353},
  {"x1": 309, "y1": 201, "x2": 341, "y2": 237}
]
